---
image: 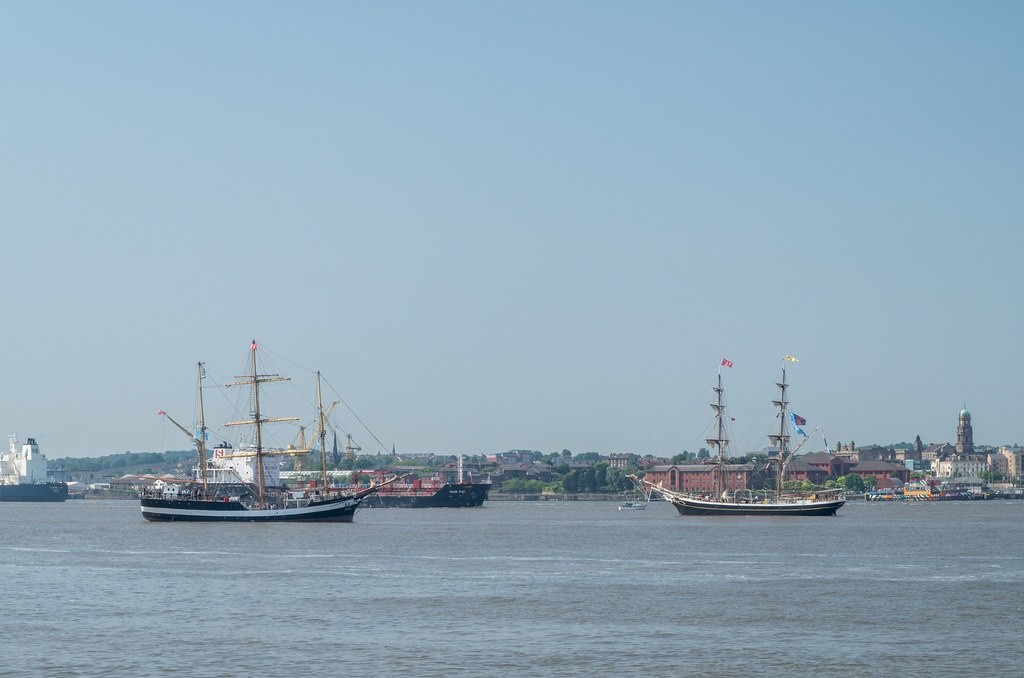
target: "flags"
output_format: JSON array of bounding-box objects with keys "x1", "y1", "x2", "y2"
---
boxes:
[
  {"x1": 790, "y1": 413, "x2": 809, "y2": 438},
  {"x1": 722, "y1": 358, "x2": 733, "y2": 367},
  {"x1": 195, "y1": 431, "x2": 209, "y2": 440},
  {"x1": 787, "y1": 356, "x2": 799, "y2": 363}
]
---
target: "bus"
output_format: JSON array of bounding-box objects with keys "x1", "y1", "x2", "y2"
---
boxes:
[{"x1": 868, "y1": 490, "x2": 905, "y2": 501}]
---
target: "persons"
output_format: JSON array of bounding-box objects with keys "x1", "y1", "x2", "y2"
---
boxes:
[
  {"x1": 815, "y1": 492, "x2": 819, "y2": 502},
  {"x1": 264, "y1": 502, "x2": 278, "y2": 510},
  {"x1": 159, "y1": 487, "x2": 163, "y2": 499},
  {"x1": 721, "y1": 489, "x2": 727, "y2": 502},
  {"x1": 754, "y1": 495, "x2": 759, "y2": 504},
  {"x1": 181, "y1": 488, "x2": 229, "y2": 502}
]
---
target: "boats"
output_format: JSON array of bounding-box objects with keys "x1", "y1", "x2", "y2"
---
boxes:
[
  {"x1": 0, "y1": 434, "x2": 70, "y2": 503},
  {"x1": 286, "y1": 370, "x2": 496, "y2": 508},
  {"x1": 618, "y1": 501, "x2": 647, "y2": 511}
]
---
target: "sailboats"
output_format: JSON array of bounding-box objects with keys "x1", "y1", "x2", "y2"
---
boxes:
[
  {"x1": 624, "y1": 351, "x2": 849, "y2": 516},
  {"x1": 136, "y1": 338, "x2": 417, "y2": 522}
]
